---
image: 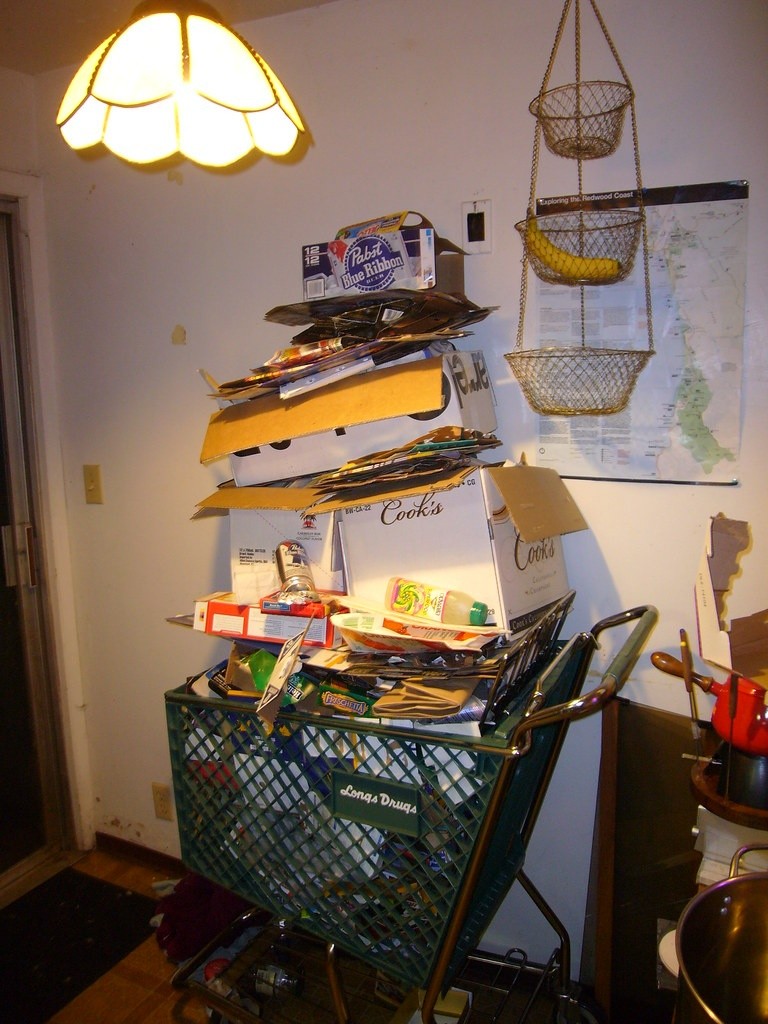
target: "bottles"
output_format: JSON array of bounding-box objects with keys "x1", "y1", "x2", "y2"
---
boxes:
[{"x1": 384, "y1": 576, "x2": 488, "y2": 626}]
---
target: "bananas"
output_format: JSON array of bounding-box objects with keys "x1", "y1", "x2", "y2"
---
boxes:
[{"x1": 525, "y1": 208, "x2": 623, "y2": 280}]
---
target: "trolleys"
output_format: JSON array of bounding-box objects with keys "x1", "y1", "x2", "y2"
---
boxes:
[{"x1": 160, "y1": 589, "x2": 659, "y2": 1024}]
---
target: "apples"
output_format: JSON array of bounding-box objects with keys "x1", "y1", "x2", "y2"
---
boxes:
[{"x1": 204, "y1": 959, "x2": 237, "y2": 988}]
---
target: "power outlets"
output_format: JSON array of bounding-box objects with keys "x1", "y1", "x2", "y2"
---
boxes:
[{"x1": 151, "y1": 782, "x2": 175, "y2": 822}]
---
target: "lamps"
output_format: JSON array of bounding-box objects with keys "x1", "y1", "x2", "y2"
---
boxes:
[{"x1": 56, "y1": 0, "x2": 306, "y2": 168}]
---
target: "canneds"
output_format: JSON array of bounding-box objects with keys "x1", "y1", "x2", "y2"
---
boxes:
[{"x1": 275, "y1": 538, "x2": 316, "y2": 591}]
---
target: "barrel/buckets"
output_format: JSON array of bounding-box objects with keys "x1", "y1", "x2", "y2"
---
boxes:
[{"x1": 674, "y1": 843, "x2": 768, "y2": 1024}]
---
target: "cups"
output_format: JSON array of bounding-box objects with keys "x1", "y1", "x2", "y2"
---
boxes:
[{"x1": 276, "y1": 539, "x2": 315, "y2": 593}]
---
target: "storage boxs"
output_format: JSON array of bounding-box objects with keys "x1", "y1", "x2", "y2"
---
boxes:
[{"x1": 164, "y1": 348, "x2": 589, "y2": 951}]
